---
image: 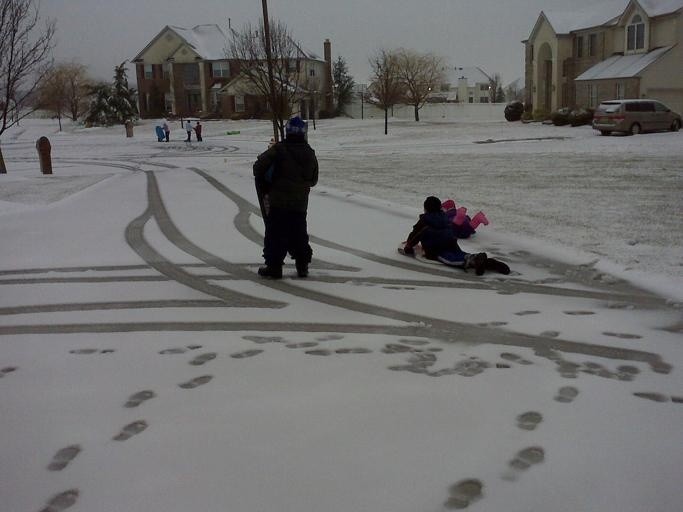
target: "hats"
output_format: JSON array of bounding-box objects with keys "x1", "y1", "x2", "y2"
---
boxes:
[
  {"x1": 286, "y1": 116, "x2": 307, "y2": 134},
  {"x1": 424, "y1": 196, "x2": 455, "y2": 212}
]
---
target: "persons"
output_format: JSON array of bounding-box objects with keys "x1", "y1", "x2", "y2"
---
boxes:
[
  {"x1": 156, "y1": 123, "x2": 170, "y2": 142},
  {"x1": 193, "y1": 121, "x2": 202, "y2": 142},
  {"x1": 185, "y1": 120, "x2": 192, "y2": 142},
  {"x1": 404, "y1": 196, "x2": 510, "y2": 276},
  {"x1": 254, "y1": 116, "x2": 319, "y2": 280}
]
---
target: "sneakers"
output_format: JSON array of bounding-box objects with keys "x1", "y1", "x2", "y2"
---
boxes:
[
  {"x1": 258, "y1": 264, "x2": 282, "y2": 279},
  {"x1": 295, "y1": 260, "x2": 308, "y2": 277},
  {"x1": 470, "y1": 252, "x2": 510, "y2": 276}
]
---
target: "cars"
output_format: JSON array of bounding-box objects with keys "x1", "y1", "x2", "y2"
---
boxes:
[{"x1": 592, "y1": 99, "x2": 682, "y2": 136}]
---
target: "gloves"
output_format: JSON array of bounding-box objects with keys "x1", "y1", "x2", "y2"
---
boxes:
[{"x1": 404, "y1": 246, "x2": 414, "y2": 254}]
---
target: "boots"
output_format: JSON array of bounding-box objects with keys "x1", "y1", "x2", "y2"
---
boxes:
[
  {"x1": 470, "y1": 212, "x2": 489, "y2": 228},
  {"x1": 454, "y1": 207, "x2": 467, "y2": 225}
]
